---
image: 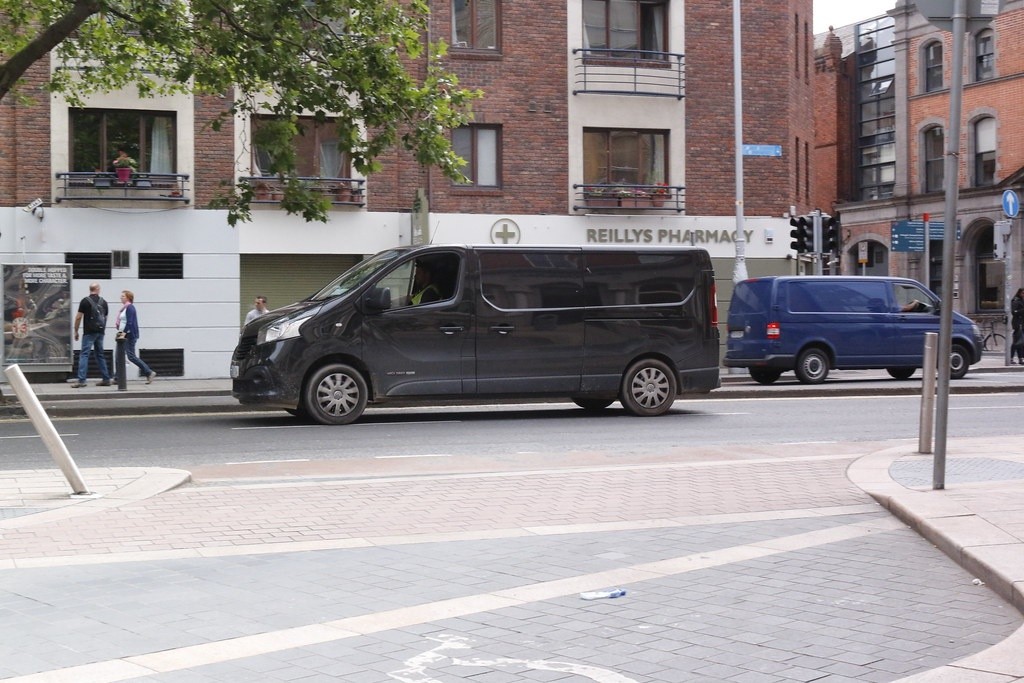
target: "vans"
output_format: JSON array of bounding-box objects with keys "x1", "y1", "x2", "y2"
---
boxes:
[
  {"x1": 230, "y1": 245, "x2": 722, "y2": 425},
  {"x1": 724, "y1": 275, "x2": 983, "y2": 386}
]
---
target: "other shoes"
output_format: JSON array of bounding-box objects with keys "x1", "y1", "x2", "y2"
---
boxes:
[
  {"x1": 96, "y1": 380, "x2": 111, "y2": 386},
  {"x1": 1019, "y1": 359, "x2": 1024, "y2": 364},
  {"x1": 145, "y1": 372, "x2": 155, "y2": 383},
  {"x1": 71, "y1": 382, "x2": 87, "y2": 388},
  {"x1": 1011, "y1": 360, "x2": 1016, "y2": 364}
]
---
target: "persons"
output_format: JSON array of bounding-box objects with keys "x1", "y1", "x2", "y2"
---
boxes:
[
  {"x1": 1010, "y1": 288, "x2": 1024, "y2": 365},
  {"x1": 70, "y1": 281, "x2": 111, "y2": 388},
  {"x1": 409, "y1": 263, "x2": 443, "y2": 306},
  {"x1": 110, "y1": 290, "x2": 157, "y2": 384},
  {"x1": 244, "y1": 296, "x2": 269, "y2": 325},
  {"x1": 899, "y1": 300, "x2": 919, "y2": 312}
]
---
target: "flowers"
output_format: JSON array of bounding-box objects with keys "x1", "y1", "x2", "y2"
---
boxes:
[
  {"x1": 586, "y1": 184, "x2": 635, "y2": 197},
  {"x1": 635, "y1": 188, "x2": 650, "y2": 197},
  {"x1": 650, "y1": 183, "x2": 668, "y2": 193},
  {"x1": 113, "y1": 151, "x2": 138, "y2": 174}
]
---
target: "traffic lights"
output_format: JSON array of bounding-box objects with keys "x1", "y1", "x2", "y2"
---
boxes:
[
  {"x1": 821, "y1": 216, "x2": 837, "y2": 255},
  {"x1": 790, "y1": 218, "x2": 805, "y2": 254},
  {"x1": 799, "y1": 216, "x2": 817, "y2": 253}
]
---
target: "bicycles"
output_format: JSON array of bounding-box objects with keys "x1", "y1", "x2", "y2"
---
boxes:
[{"x1": 982, "y1": 320, "x2": 1006, "y2": 351}]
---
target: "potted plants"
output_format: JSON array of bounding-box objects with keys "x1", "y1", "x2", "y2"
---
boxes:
[{"x1": 253, "y1": 180, "x2": 364, "y2": 203}]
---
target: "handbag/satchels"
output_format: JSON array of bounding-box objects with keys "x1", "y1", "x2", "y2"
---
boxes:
[{"x1": 115, "y1": 332, "x2": 127, "y2": 341}]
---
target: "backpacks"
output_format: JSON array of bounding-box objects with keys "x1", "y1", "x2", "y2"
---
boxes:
[{"x1": 84, "y1": 297, "x2": 106, "y2": 329}]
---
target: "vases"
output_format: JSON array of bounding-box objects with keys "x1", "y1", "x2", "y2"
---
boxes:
[
  {"x1": 586, "y1": 195, "x2": 618, "y2": 207},
  {"x1": 652, "y1": 192, "x2": 667, "y2": 206},
  {"x1": 618, "y1": 196, "x2": 635, "y2": 207},
  {"x1": 115, "y1": 168, "x2": 132, "y2": 182},
  {"x1": 636, "y1": 196, "x2": 651, "y2": 207}
]
